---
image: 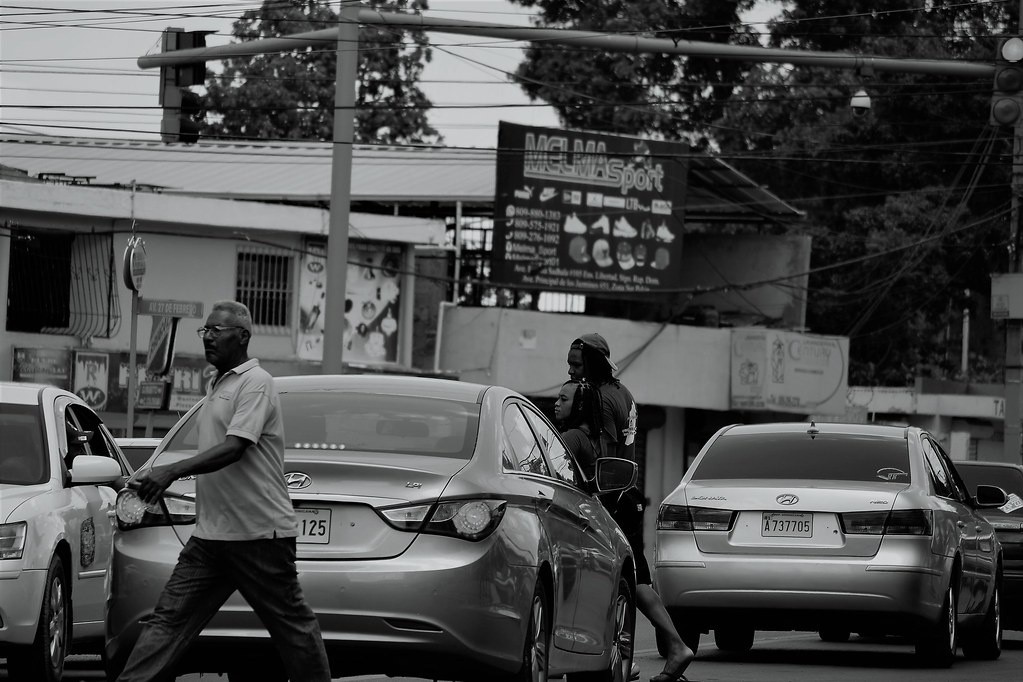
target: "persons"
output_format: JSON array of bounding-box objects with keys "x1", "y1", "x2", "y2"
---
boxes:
[
  {"x1": 555, "y1": 333, "x2": 694, "y2": 682},
  {"x1": 117, "y1": 302, "x2": 331, "y2": 682}
]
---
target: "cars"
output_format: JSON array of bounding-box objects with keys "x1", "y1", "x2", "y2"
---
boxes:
[
  {"x1": 0, "y1": 381, "x2": 142, "y2": 682},
  {"x1": 946, "y1": 460, "x2": 1023, "y2": 625},
  {"x1": 106, "y1": 373, "x2": 641, "y2": 682},
  {"x1": 110, "y1": 437, "x2": 162, "y2": 477},
  {"x1": 654, "y1": 418, "x2": 1007, "y2": 669}
]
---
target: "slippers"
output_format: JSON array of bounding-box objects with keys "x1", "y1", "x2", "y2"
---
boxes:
[{"x1": 649, "y1": 672, "x2": 700, "y2": 682}]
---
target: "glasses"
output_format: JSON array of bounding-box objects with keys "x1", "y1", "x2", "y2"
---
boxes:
[{"x1": 196, "y1": 326, "x2": 245, "y2": 338}]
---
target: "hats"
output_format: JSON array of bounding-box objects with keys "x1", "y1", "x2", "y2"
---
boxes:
[{"x1": 571, "y1": 333, "x2": 618, "y2": 371}]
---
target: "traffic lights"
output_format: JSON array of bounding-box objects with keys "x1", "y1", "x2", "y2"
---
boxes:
[{"x1": 166, "y1": 25, "x2": 213, "y2": 145}]
